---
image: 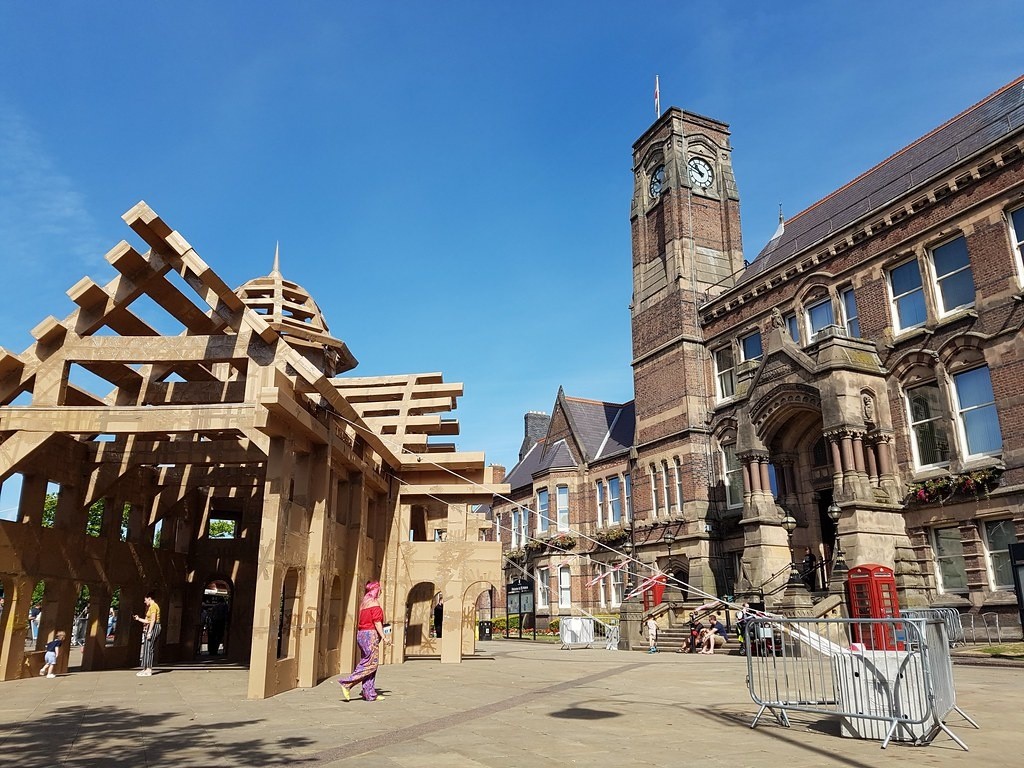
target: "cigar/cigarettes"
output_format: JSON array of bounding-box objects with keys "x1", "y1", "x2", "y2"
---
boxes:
[{"x1": 391, "y1": 644, "x2": 395, "y2": 646}]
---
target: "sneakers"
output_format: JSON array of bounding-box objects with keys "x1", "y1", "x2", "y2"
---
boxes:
[
  {"x1": 47, "y1": 673, "x2": 56, "y2": 679},
  {"x1": 375, "y1": 695, "x2": 386, "y2": 701},
  {"x1": 137, "y1": 670, "x2": 152, "y2": 676},
  {"x1": 340, "y1": 684, "x2": 350, "y2": 700},
  {"x1": 39, "y1": 670, "x2": 46, "y2": 676}
]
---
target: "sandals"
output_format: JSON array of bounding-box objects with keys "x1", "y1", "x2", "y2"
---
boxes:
[
  {"x1": 685, "y1": 649, "x2": 692, "y2": 653},
  {"x1": 676, "y1": 648, "x2": 684, "y2": 652}
]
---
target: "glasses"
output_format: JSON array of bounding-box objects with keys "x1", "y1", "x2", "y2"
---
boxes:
[{"x1": 709, "y1": 619, "x2": 712, "y2": 621}]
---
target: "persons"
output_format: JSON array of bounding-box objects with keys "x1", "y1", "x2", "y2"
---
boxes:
[
  {"x1": 647, "y1": 614, "x2": 660, "y2": 651},
  {"x1": 337, "y1": 581, "x2": 392, "y2": 702},
  {"x1": 434, "y1": 597, "x2": 443, "y2": 638},
  {"x1": 0, "y1": 592, "x2": 229, "y2": 677},
  {"x1": 697, "y1": 615, "x2": 728, "y2": 655},
  {"x1": 803, "y1": 546, "x2": 816, "y2": 592},
  {"x1": 675, "y1": 620, "x2": 708, "y2": 653}
]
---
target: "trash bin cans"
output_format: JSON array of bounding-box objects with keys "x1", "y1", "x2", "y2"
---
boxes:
[{"x1": 478, "y1": 620, "x2": 493, "y2": 641}]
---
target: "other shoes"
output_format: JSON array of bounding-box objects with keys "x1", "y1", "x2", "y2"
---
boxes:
[
  {"x1": 648, "y1": 649, "x2": 654, "y2": 654},
  {"x1": 698, "y1": 651, "x2": 706, "y2": 654},
  {"x1": 702, "y1": 651, "x2": 714, "y2": 654},
  {"x1": 652, "y1": 648, "x2": 657, "y2": 651}
]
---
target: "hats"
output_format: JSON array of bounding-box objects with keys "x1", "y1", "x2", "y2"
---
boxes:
[{"x1": 367, "y1": 581, "x2": 380, "y2": 597}]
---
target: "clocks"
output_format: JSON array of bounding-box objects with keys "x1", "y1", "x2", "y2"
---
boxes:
[
  {"x1": 649, "y1": 164, "x2": 665, "y2": 198},
  {"x1": 687, "y1": 157, "x2": 715, "y2": 188}
]
